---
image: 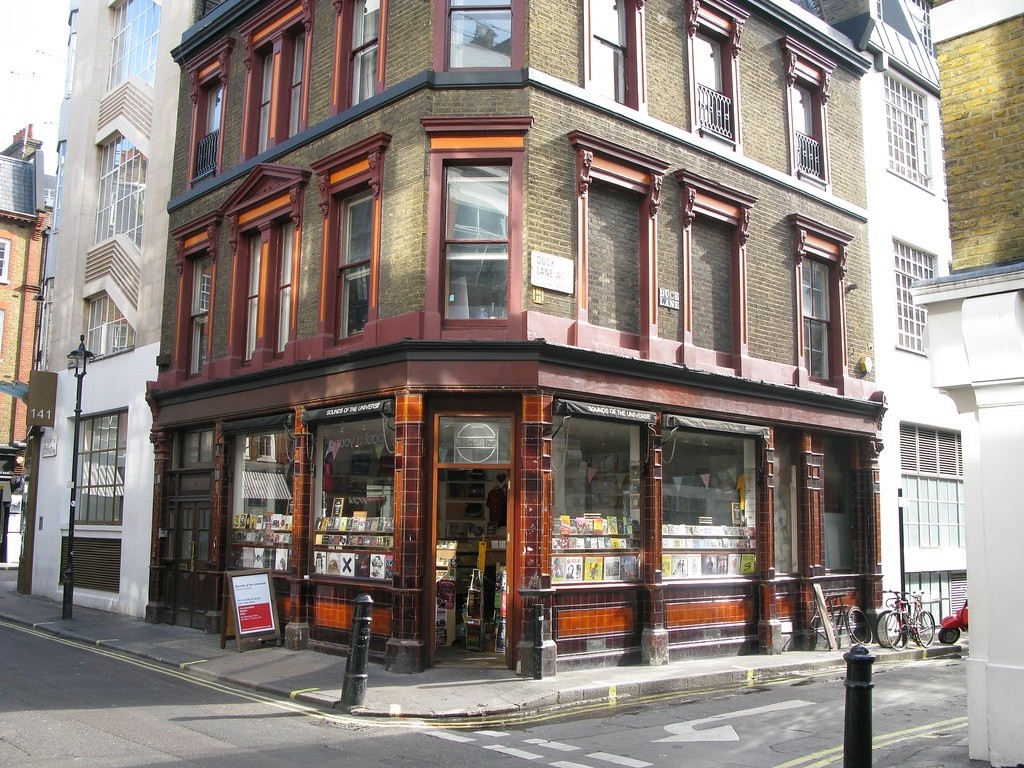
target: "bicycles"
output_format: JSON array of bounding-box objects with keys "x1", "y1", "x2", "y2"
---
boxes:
[{"x1": 872, "y1": 589, "x2": 936, "y2": 652}]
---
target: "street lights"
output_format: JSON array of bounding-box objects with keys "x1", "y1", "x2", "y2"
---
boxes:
[{"x1": 62, "y1": 334, "x2": 96, "y2": 620}]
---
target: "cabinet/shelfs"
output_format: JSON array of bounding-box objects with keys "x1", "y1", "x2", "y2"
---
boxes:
[
  {"x1": 464, "y1": 537, "x2": 507, "y2": 651},
  {"x1": 437, "y1": 462, "x2": 491, "y2": 538},
  {"x1": 279, "y1": 473, "x2": 394, "y2": 517},
  {"x1": 352, "y1": 443, "x2": 395, "y2": 476},
  {"x1": 663, "y1": 495, "x2": 707, "y2": 526},
  {"x1": 435, "y1": 539, "x2": 480, "y2": 646}
]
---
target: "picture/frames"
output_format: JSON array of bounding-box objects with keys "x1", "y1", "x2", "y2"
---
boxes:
[
  {"x1": 604, "y1": 455, "x2": 618, "y2": 473},
  {"x1": 457, "y1": 448, "x2": 473, "y2": 470},
  {"x1": 578, "y1": 456, "x2": 592, "y2": 474}
]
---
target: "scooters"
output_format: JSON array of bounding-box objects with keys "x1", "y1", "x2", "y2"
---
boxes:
[{"x1": 937, "y1": 599, "x2": 968, "y2": 644}]
